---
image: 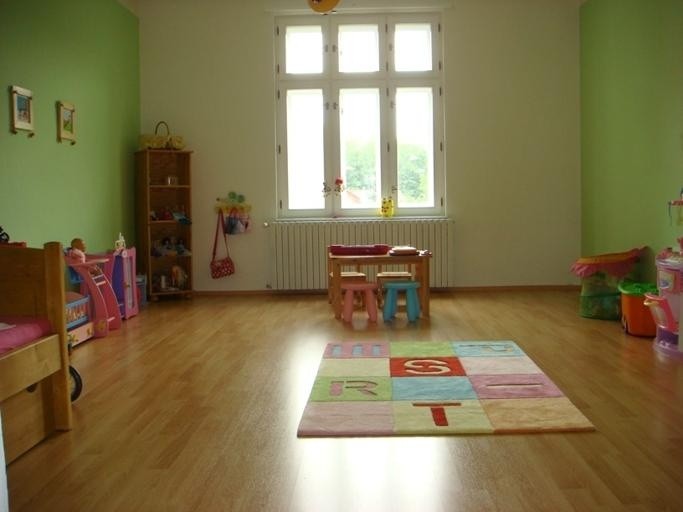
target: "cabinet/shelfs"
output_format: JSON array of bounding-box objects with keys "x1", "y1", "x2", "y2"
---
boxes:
[{"x1": 134, "y1": 149, "x2": 194, "y2": 303}]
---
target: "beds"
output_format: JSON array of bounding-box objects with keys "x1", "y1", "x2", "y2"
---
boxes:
[{"x1": 1, "y1": 241, "x2": 73, "y2": 469}]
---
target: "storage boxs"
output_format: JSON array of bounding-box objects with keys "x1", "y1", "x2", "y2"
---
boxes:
[{"x1": 616, "y1": 282, "x2": 658, "y2": 337}]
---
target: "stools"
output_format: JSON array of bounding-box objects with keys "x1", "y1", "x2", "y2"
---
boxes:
[{"x1": 328, "y1": 272, "x2": 421, "y2": 323}]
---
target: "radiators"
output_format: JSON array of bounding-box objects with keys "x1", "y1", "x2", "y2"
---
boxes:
[{"x1": 269, "y1": 219, "x2": 456, "y2": 291}]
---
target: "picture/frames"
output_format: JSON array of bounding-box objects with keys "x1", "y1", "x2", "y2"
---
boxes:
[
  {"x1": 12, "y1": 86, "x2": 35, "y2": 131},
  {"x1": 58, "y1": 100, "x2": 77, "y2": 140}
]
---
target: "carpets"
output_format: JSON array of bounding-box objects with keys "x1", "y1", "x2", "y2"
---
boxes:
[{"x1": 297, "y1": 340, "x2": 597, "y2": 435}]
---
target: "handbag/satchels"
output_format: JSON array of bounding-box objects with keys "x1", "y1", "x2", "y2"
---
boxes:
[
  {"x1": 210, "y1": 258, "x2": 234, "y2": 278},
  {"x1": 225, "y1": 206, "x2": 252, "y2": 234}
]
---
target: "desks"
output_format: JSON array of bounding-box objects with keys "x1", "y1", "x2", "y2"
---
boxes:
[{"x1": 328, "y1": 252, "x2": 432, "y2": 319}]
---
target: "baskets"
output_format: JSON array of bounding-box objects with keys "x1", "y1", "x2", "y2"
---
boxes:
[{"x1": 138, "y1": 121, "x2": 185, "y2": 151}]
